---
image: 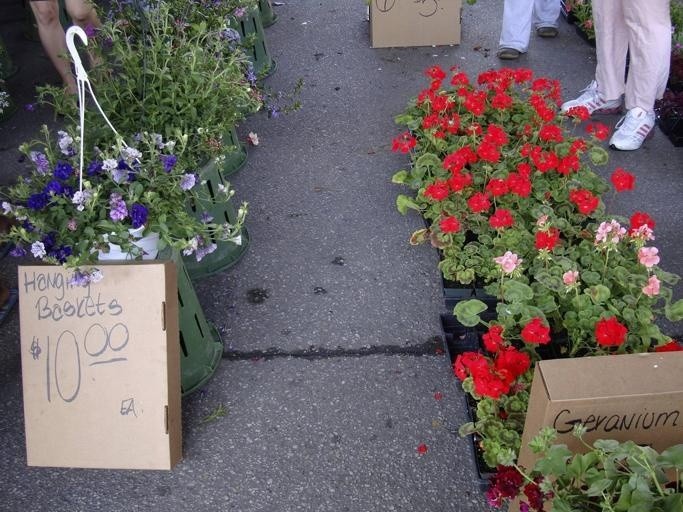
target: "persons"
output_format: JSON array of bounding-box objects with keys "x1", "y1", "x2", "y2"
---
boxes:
[
  {"x1": 561, "y1": 1, "x2": 673, "y2": 151},
  {"x1": 28, "y1": 0, "x2": 104, "y2": 116},
  {"x1": 497, "y1": 0, "x2": 561, "y2": 60}
]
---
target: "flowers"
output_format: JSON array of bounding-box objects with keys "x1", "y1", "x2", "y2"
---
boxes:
[
  {"x1": 0, "y1": 1, "x2": 305, "y2": 288},
  {"x1": 389, "y1": 66, "x2": 682, "y2": 511}
]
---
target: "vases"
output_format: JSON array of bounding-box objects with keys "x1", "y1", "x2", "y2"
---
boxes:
[{"x1": 152, "y1": 244, "x2": 225, "y2": 400}]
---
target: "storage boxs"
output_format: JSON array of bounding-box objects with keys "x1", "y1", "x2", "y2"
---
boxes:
[
  {"x1": 368, "y1": 0, "x2": 461, "y2": 49},
  {"x1": 17, "y1": 261, "x2": 183, "y2": 470}
]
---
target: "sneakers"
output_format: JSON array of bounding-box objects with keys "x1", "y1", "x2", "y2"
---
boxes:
[
  {"x1": 608, "y1": 107, "x2": 656, "y2": 152},
  {"x1": 560, "y1": 78, "x2": 623, "y2": 115},
  {"x1": 537, "y1": 26, "x2": 559, "y2": 37},
  {"x1": 497, "y1": 47, "x2": 520, "y2": 59}
]
